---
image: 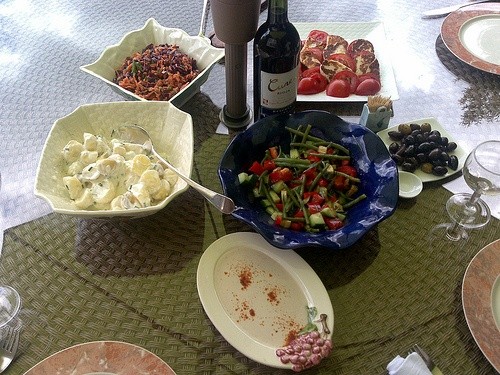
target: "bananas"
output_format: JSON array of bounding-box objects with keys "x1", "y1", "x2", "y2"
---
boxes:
[{"x1": 62, "y1": 133, "x2": 178, "y2": 209}]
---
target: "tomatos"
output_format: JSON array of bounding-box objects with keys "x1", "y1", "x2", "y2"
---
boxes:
[{"x1": 297, "y1": 30, "x2": 381, "y2": 98}]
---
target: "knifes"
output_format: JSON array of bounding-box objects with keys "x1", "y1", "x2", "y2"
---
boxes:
[{"x1": 422, "y1": 0, "x2": 499, "y2": 15}]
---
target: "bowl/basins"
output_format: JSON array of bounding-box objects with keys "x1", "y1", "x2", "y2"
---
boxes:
[
  {"x1": 33, "y1": 101, "x2": 194, "y2": 221},
  {"x1": 397, "y1": 171, "x2": 423, "y2": 198},
  {"x1": 77, "y1": 19, "x2": 226, "y2": 109}
]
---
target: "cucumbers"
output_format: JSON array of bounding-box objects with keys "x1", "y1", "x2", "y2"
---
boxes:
[{"x1": 239, "y1": 146, "x2": 336, "y2": 228}]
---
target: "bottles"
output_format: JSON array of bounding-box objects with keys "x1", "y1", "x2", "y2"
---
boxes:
[{"x1": 254, "y1": 0, "x2": 300, "y2": 125}]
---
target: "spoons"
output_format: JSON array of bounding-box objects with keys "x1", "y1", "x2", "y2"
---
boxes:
[{"x1": 116, "y1": 124, "x2": 234, "y2": 214}]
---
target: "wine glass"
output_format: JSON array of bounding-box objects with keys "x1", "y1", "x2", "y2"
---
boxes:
[
  {"x1": 0, "y1": 282, "x2": 20, "y2": 329},
  {"x1": 446, "y1": 140, "x2": 500, "y2": 228}
]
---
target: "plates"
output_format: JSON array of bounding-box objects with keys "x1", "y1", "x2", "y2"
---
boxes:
[
  {"x1": 218, "y1": 110, "x2": 399, "y2": 251},
  {"x1": 196, "y1": 231, "x2": 334, "y2": 369},
  {"x1": 461, "y1": 239, "x2": 500, "y2": 374},
  {"x1": 286, "y1": 22, "x2": 399, "y2": 102},
  {"x1": 440, "y1": 2, "x2": 500, "y2": 76},
  {"x1": 22, "y1": 341, "x2": 179, "y2": 375},
  {"x1": 376, "y1": 117, "x2": 467, "y2": 183}
]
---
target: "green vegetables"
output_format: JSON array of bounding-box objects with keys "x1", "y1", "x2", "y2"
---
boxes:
[
  {"x1": 258, "y1": 125, "x2": 366, "y2": 225},
  {"x1": 132, "y1": 59, "x2": 174, "y2": 91}
]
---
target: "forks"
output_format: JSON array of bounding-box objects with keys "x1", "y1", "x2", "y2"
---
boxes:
[
  {"x1": 0, "y1": 326, "x2": 20, "y2": 375},
  {"x1": 407, "y1": 344, "x2": 444, "y2": 375}
]
